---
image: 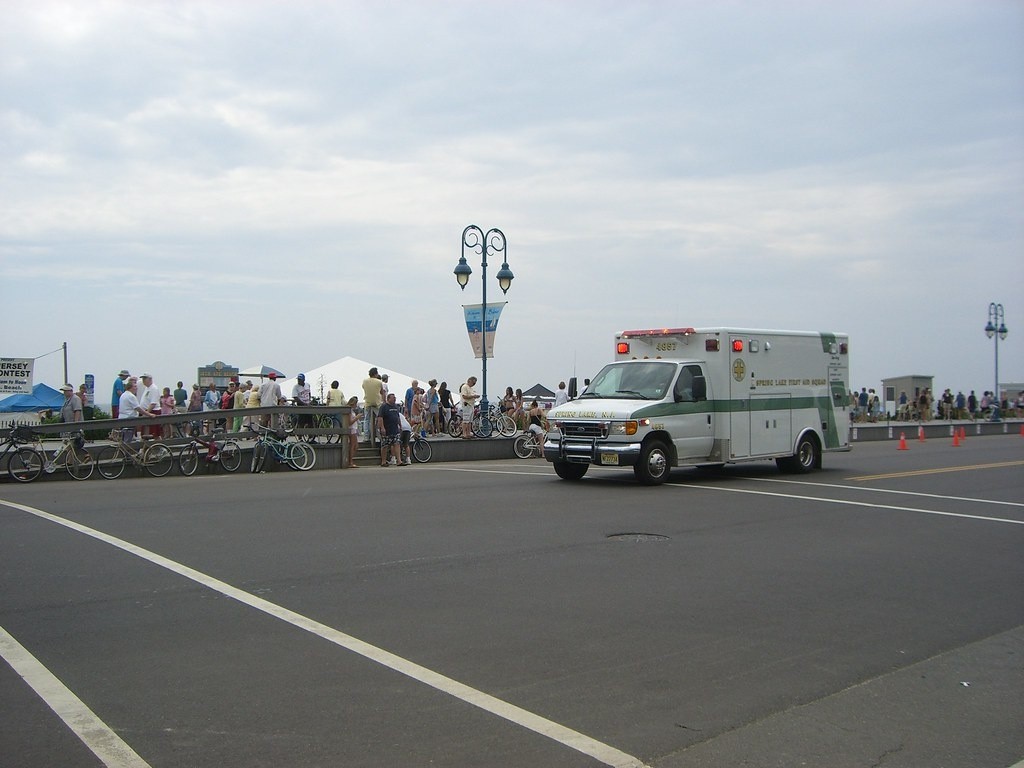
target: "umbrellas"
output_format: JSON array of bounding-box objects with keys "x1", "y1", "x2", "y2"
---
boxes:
[{"x1": 238, "y1": 365, "x2": 286, "y2": 384}]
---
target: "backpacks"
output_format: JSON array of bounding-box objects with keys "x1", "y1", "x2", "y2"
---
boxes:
[{"x1": 218, "y1": 390, "x2": 231, "y2": 409}]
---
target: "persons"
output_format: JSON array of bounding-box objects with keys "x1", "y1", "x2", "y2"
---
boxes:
[
  {"x1": 61, "y1": 384, "x2": 92, "y2": 466},
  {"x1": 73, "y1": 384, "x2": 89, "y2": 407},
  {"x1": 848, "y1": 387, "x2": 1024, "y2": 423},
  {"x1": 108, "y1": 366, "x2": 590, "y2": 469}
]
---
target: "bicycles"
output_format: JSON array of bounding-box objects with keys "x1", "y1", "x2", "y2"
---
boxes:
[
  {"x1": 379, "y1": 422, "x2": 433, "y2": 464},
  {"x1": 243, "y1": 422, "x2": 317, "y2": 474},
  {"x1": 96, "y1": 428, "x2": 175, "y2": 481},
  {"x1": 26, "y1": 430, "x2": 94, "y2": 481},
  {"x1": 293, "y1": 397, "x2": 343, "y2": 446},
  {"x1": 513, "y1": 421, "x2": 548, "y2": 459},
  {"x1": 447, "y1": 396, "x2": 517, "y2": 439},
  {"x1": 178, "y1": 420, "x2": 243, "y2": 477},
  {"x1": 0, "y1": 424, "x2": 44, "y2": 483}
]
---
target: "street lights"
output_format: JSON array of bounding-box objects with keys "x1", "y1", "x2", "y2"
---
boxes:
[
  {"x1": 453, "y1": 224, "x2": 515, "y2": 436},
  {"x1": 985, "y1": 302, "x2": 1008, "y2": 421}
]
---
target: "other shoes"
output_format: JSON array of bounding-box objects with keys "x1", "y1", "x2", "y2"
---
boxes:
[
  {"x1": 349, "y1": 464, "x2": 357, "y2": 467},
  {"x1": 61, "y1": 462, "x2": 73, "y2": 467},
  {"x1": 309, "y1": 440, "x2": 318, "y2": 444},
  {"x1": 423, "y1": 430, "x2": 453, "y2": 438},
  {"x1": 381, "y1": 454, "x2": 412, "y2": 467},
  {"x1": 463, "y1": 434, "x2": 477, "y2": 439},
  {"x1": 136, "y1": 454, "x2": 151, "y2": 461},
  {"x1": 84, "y1": 454, "x2": 91, "y2": 463},
  {"x1": 159, "y1": 449, "x2": 168, "y2": 456},
  {"x1": 364, "y1": 436, "x2": 370, "y2": 442}
]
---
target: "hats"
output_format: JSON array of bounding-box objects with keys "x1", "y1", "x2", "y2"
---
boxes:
[
  {"x1": 229, "y1": 382, "x2": 235, "y2": 385},
  {"x1": 382, "y1": 374, "x2": 390, "y2": 380},
  {"x1": 118, "y1": 370, "x2": 131, "y2": 376},
  {"x1": 59, "y1": 385, "x2": 73, "y2": 391},
  {"x1": 268, "y1": 372, "x2": 277, "y2": 378},
  {"x1": 297, "y1": 374, "x2": 305, "y2": 380},
  {"x1": 193, "y1": 383, "x2": 200, "y2": 389},
  {"x1": 139, "y1": 372, "x2": 153, "y2": 379}
]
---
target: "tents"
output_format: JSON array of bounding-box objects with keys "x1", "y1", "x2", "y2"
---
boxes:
[
  {"x1": 277, "y1": 355, "x2": 460, "y2": 417},
  {"x1": 0, "y1": 382, "x2": 66, "y2": 443},
  {"x1": 508, "y1": 383, "x2": 558, "y2": 404}
]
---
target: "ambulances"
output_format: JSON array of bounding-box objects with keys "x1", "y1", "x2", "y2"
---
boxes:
[{"x1": 543, "y1": 327, "x2": 853, "y2": 486}]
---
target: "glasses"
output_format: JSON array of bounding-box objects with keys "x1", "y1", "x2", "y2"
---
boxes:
[{"x1": 229, "y1": 385, "x2": 234, "y2": 387}]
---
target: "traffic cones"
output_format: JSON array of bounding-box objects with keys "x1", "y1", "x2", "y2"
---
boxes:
[
  {"x1": 917, "y1": 428, "x2": 927, "y2": 442},
  {"x1": 897, "y1": 432, "x2": 910, "y2": 450},
  {"x1": 1020, "y1": 425, "x2": 1024, "y2": 438},
  {"x1": 960, "y1": 426, "x2": 967, "y2": 440},
  {"x1": 951, "y1": 430, "x2": 961, "y2": 446}
]
